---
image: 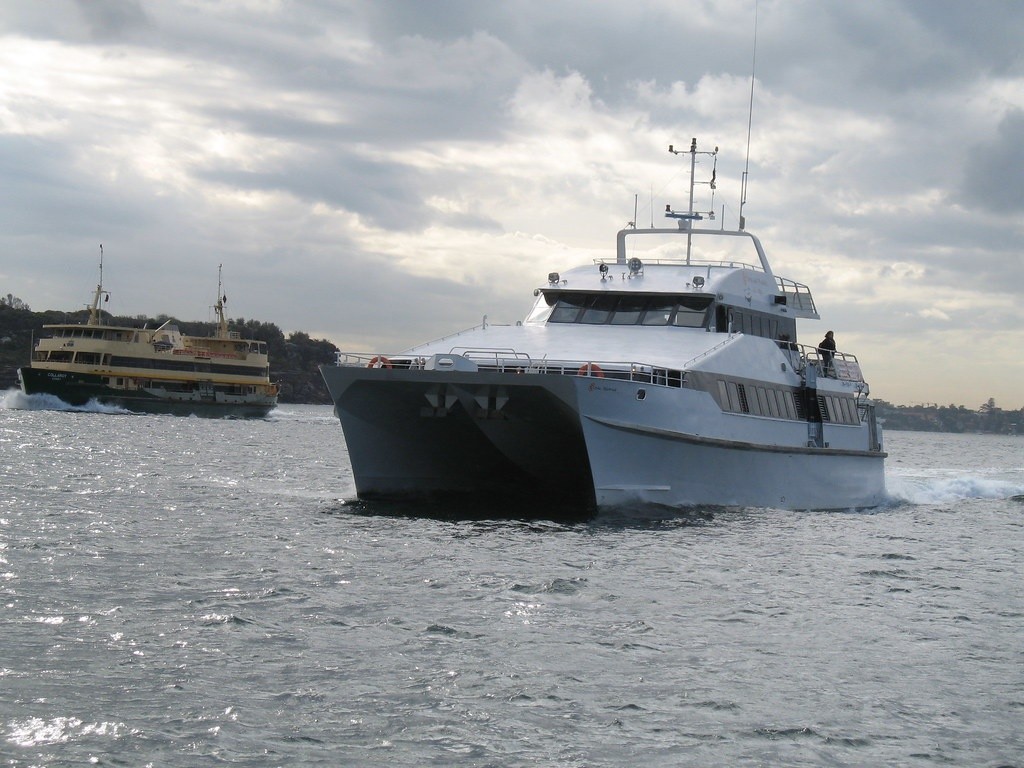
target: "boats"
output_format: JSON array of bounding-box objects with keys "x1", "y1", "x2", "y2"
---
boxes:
[
  {"x1": 314, "y1": 2, "x2": 888, "y2": 516},
  {"x1": 18, "y1": 246, "x2": 284, "y2": 419}
]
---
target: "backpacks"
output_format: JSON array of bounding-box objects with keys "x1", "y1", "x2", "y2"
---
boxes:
[{"x1": 818, "y1": 342, "x2": 823, "y2": 354}]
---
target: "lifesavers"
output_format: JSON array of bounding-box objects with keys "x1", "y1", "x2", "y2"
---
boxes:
[
  {"x1": 367, "y1": 357, "x2": 393, "y2": 369},
  {"x1": 577, "y1": 364, "x2": 605, "y2": 378}
]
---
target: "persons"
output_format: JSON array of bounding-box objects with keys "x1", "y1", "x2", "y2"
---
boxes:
[{"x1": 818, "y1": 331, "x2": 837, "y2": 378}]
